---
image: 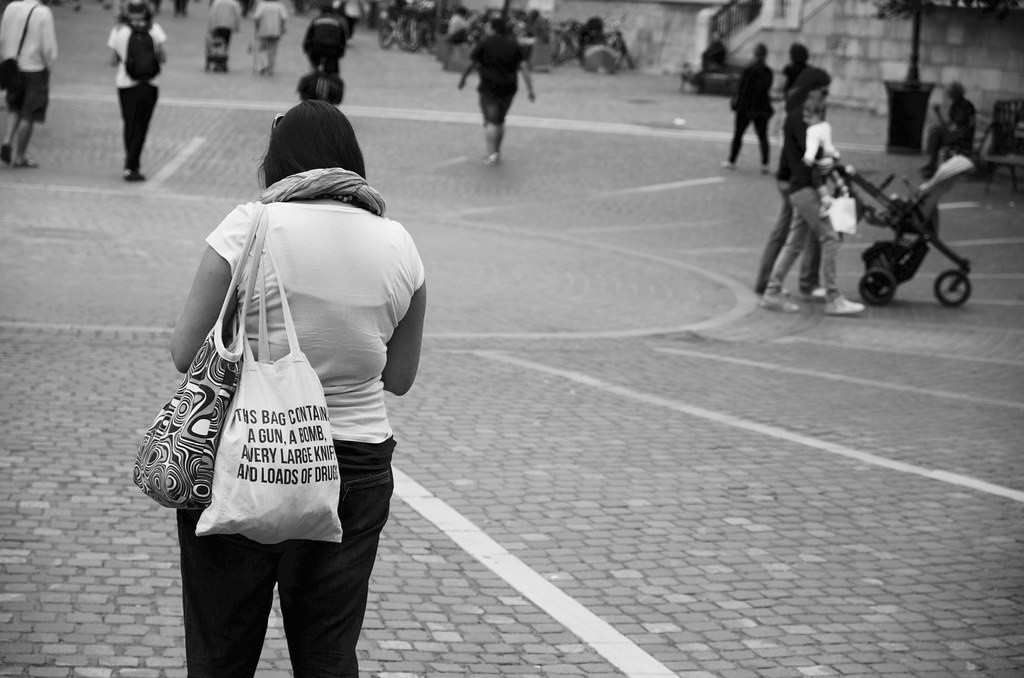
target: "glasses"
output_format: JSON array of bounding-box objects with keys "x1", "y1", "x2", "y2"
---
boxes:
[{"x1": 272, "y1": 113, "x2": 285, "y2": 131}]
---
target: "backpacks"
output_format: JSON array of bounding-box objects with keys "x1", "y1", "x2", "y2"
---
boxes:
[{"x1": 119, "y1": 21, "x2": 160, "y2": 80}]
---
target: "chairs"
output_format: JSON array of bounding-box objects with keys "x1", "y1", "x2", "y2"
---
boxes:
[{"x1": 940, "y1": 98, "x2": 1024, "y2": 195}]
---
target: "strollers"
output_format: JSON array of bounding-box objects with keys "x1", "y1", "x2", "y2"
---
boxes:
[{"x1": 826, "y1": 153, "x2": 978, "y2": 308}]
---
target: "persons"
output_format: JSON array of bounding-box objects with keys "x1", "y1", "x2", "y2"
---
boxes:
[
  {"x1": 755, "y1": 66, "x2": 865, "y2": 315},
  {"x1": 0, "y1": 0, "x2": 58, "y2": 170},
  {"x1": 921, "y1": 81, "x2": 976, "y2": 178},
  {"x1": 337, "y1": 0, "x2": 369, "y2": 40},
  {"x1": 208, "y1": 0, "x2": 242, "y2": 71},
  {"x1": 457, "y1": 19, "x2": 536, "y2": 164},
  {"x1": 302, "y1": 6, "x2": 348, "y2": 74},
  {"x1": 781, "y1": 44, "x2": 811, "y2": 92},
  {"x1": 447, "y1": 8, "x2": 468, "y2": 45},
  {"x1": 104, "y1": 0, "x2": 168, "y2": 183},
  {"x1": 722, "y1": 45, "x2": 775, "y2": 173},
  {"x1": 253, "y1": 0, "x2": 289, "y2": 75},
  {"x1": 169, "y1": 99, "x2": 426, "y2": 678},
  {"x1": 611, "y1": 32, "x2": 634, "y2": 69},
  {"x1": 679, "y1": 30, "x2": 728, "y2": 95}
]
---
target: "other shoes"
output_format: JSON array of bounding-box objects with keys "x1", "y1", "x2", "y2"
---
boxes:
[
  {"x1": 758, "y1": 295, "x2": 801, "y2": 314},
  {"x1": 807, "y1": 287, "x2": 827, "y2": 299},
  {"x1": 1, "y1": 144, "x2": 12, "y2": 166},
  {"x1": 761, "y1": 166, "x2": 768, "y2": 175},
  {"x1": 826, "y1": 296, "x2": 865, "y2": 315},
  {"x1": 819, "y1": 197, "x2": 834, "y2": 214},
  {"x1": 123, "y1": 169, "x2": 144, "y2": 180},
  {"x1": 721, "y1": 161, "x2": 735, "y2": 169},
  {"x1": 486, "y1": 152, "x2": 499, "y2": 162},
  {"x1": 12, "y1": 159, "x2": 38, "y2": 169}
]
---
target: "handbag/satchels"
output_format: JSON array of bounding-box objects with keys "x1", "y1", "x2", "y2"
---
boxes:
[
  {"x1": 195, "y1": 203, "x2": 344, "y2": 544},
  {"x1": 0, "y1": 59, "x2": 23, "y2": 89},
  {"x1": 132, "y1": 205, "x2": 268, "y2": 509}
]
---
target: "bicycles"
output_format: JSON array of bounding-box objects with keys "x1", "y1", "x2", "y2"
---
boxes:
[
  {"x1": 548, "y1": 17, "x2": 636, "y2": 70},
  {"x1": 374, "y1": 0, "x2": 428, "y2": 52}
]
